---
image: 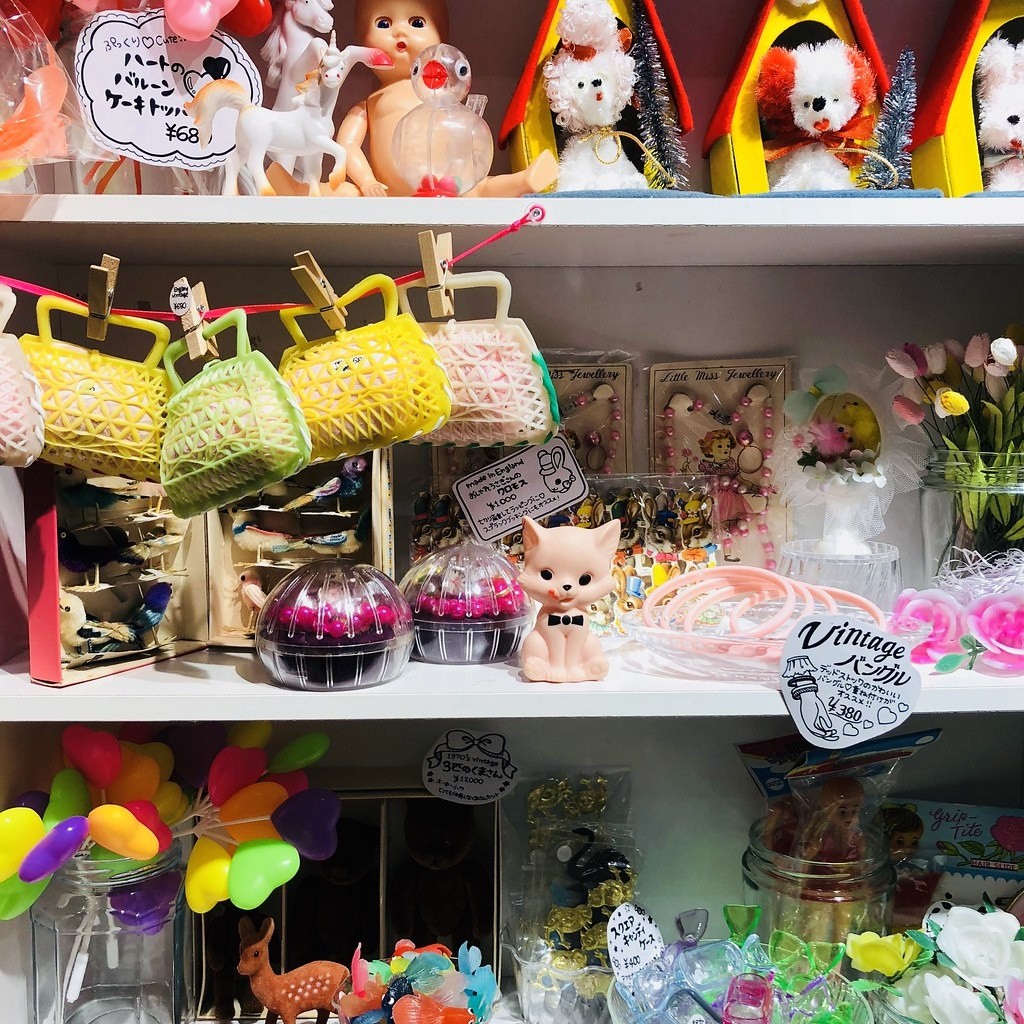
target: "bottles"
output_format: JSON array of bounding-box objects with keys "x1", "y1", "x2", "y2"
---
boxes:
[{"x1": 28, "y1": 828, "x2": 190, "y2": 1024}]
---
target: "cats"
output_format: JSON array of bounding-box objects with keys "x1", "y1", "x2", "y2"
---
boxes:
[{"x1": 519, "y1": 513, "x2": 622, "y2": 684}]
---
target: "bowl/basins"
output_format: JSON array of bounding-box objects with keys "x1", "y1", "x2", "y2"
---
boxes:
[
  {"x1": 606, "y1": 938, "x2": 874, "y2": 1024},
  {"x1": 499, "y1": 921, "x2": 613, "y2": 1024},
  {"x1": 777, "y1": 539, "x2": 905, "y2": 611}
]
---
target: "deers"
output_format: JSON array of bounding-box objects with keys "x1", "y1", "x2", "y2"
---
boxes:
[{"x1": 236, "y1": 916, "x2": 349, "y2": 1024}]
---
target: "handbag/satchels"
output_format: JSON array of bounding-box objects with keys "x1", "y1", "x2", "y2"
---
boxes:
[
  {"x1": 161, "y1": 309, "x2": 312, "y2": 519},
  {"x1": 17, "y1": 296, "x2": 172, "y2": 484},
  {"x1": 0, "y1": 285, "x2": 45, "y2": 467},
  {"x1": 398, "y1": 271, "x2": 560, "y2": 448},
  {"x1": 277, "y1": 274, "x2": 455, "y2": 467}
]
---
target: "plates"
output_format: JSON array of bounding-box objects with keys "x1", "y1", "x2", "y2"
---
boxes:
[{"x1": 619, "y1": 602, "x2": 933, "y2": 678}]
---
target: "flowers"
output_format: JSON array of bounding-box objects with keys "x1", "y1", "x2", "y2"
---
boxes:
[
  {"x1": 783, "y1": 366, "x2": 890, "y2": 488},
  {"x1": 841, "y1": 890, "x2": 1024, "y2": 1024},
  {"x1": 887, "y1": 585, "x2": 1023, "y2": 679},
  {"x1": 882, "y1": 319, "x2": 1024, "y2": 453}
]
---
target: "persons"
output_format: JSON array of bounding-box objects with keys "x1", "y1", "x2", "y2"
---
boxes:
[{"x1": 265, "y1": 0, "x2": 565, "y2": 201}]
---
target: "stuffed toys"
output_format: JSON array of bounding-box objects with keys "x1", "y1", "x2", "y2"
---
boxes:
[
  {"x1": 972, "y1": 35, "x2": 1023, "y2": 191},
  {"x1": 756, "y1": 42, "x2": 878, "y2": 190},
  {"x1": 548, "y1": 0, "x2": 649, "y2": 196}
]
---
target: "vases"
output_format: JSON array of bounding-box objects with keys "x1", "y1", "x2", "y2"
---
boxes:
[{"x1": 917, "y1": 462, "x2": 1024, "y2": 576}]
